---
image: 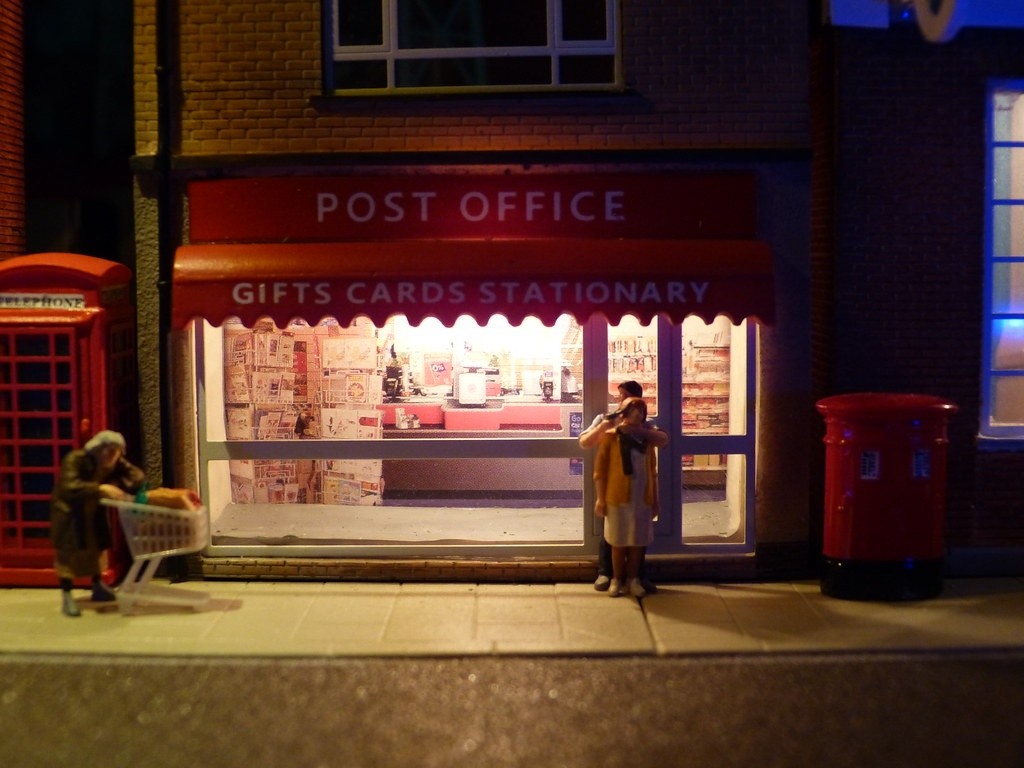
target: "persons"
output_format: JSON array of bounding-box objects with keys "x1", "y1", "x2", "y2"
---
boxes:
[
  {"x1": 579, "y1": 381, "x2": 668, "y2": 596},
  {"x1": 55, "y1": 431, "x2": 143, "y2": 614}
]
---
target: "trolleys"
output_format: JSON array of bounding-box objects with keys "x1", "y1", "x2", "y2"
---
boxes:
[{"x1": 100, "y1": 499, "x2": 207, "y2": 615}]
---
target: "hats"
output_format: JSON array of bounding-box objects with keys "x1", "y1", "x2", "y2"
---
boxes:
[{"x1": 618, "y1": 381, "x2": 642, "y2": 398}]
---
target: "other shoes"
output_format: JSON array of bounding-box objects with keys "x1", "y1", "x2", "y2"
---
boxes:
[
  {"x1": 638, "y1": 576, "x2": 657, "y2": 593},
  {"x1": 630, "y1": 583, "x2": 646, "y2": 597},
  {"x1": 607, "y1": 578, "x2": 621, "y2": 596},
  {"x1": 63, "y1": 591, "x2": 79, "y2": 615},
  {"x1": 91, "y1": 580, "x2": 116, "y2": 601},
  {"x1": 595, "y1": 575, "x2": 610, "y2": 591}
]
